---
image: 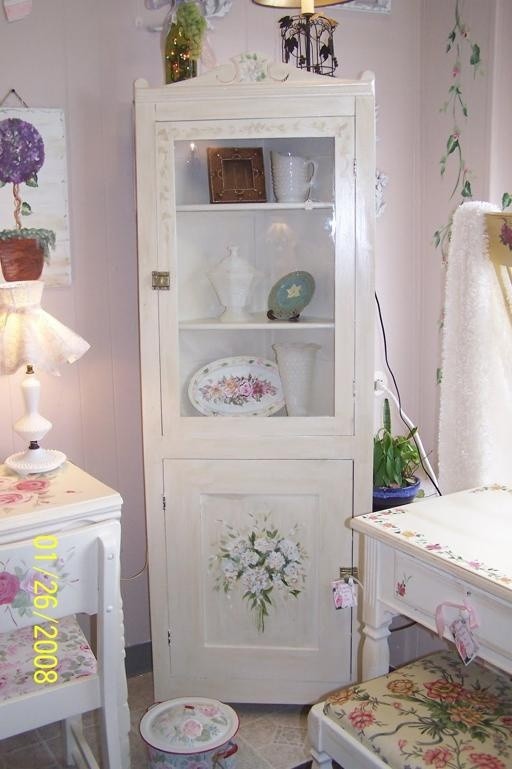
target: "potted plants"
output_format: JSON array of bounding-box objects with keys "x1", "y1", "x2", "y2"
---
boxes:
[{"x1": 373, "y1": 398, "x2": 434, "y2": 511}]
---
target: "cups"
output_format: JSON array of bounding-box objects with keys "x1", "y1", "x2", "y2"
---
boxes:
[
  {"x1": 273, "y1": 343, "x2": 322, "y2": 416},
  {"x1": 271, "y1": 150, "x2": 318, "y2": 203}
]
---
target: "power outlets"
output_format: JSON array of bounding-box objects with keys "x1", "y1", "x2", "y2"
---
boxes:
[{"x1": 374, "y1": 370, "x2": 388, "y2": 397}]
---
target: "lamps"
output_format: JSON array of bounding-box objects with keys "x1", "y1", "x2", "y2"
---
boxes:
[
  {"x1": 0, "y1": 279, "x2": 91, "y2": 473},
  {"x1": 256, "y1": 0, "x2": 354, "y2": 78}
]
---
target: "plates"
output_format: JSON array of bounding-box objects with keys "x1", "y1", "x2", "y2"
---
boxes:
[
  {"x1": 267, "y1": 271, "x2": 316, "y2": 320},
  {"x1": 188, "y1": 355, "x2": 286, "y2": 416}
]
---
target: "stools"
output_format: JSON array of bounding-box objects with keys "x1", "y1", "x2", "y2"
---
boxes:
[{"x1": 308, "y1": 647, "x2": 511, "y2": 769}]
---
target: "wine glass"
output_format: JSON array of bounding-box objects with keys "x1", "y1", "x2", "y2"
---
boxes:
[{"x1": 206, "y1": 244, "x2": 257, "y2": 322}]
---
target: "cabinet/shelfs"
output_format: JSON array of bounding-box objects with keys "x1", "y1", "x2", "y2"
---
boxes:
[
  {"x1": 0, "y1": 463, "x2": 132, "y2": 769},
  {"x1": 133, "y1": 49, "x2": 378, "y2": 707}
]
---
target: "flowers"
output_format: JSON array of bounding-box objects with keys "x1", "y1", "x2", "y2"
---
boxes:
[
  {"x1": 199, "y1": 372, "x2": 278, "y2": 408},
  {"x1": 204, "y1": 509, "x2": 311, "y2": 634},
  {"x1": 0, "y1": 119, "x2": 56, "y2": 264}
]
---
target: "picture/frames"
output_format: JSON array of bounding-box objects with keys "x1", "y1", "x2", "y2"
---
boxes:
[{"x1": 207, "y1": 146, "x2": 268, "y2": 203}]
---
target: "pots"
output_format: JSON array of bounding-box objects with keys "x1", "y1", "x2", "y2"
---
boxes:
[{"x1": 139, "y1": 696, "x2": 240, "y2": 769}]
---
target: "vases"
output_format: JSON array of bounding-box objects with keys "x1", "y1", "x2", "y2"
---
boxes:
[{"x1": 0, "y1": 238, "x2": 45, "y2": 282}]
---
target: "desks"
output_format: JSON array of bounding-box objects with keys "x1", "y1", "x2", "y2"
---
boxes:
[{"x1": 349, "y1": 482, "x2": 512, "y2": 683}]
